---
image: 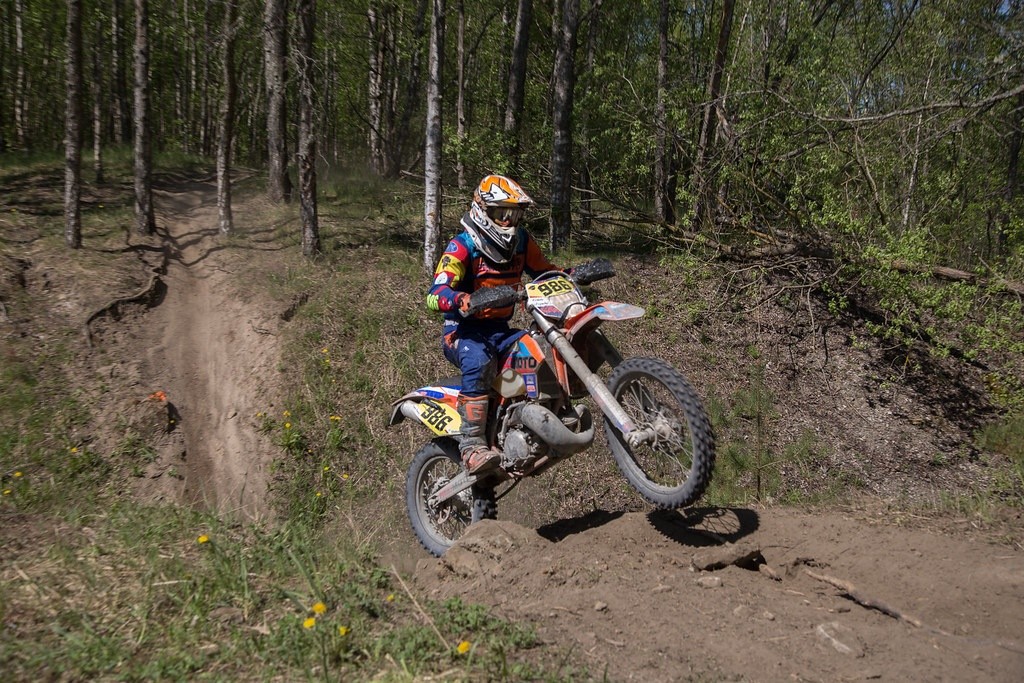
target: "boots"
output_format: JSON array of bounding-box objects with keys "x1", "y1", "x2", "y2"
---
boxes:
[{"x1": 456, "y1": 391, "x2": 501, "y2": 476}]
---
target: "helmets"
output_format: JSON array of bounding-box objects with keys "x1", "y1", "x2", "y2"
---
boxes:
[{"x1": 470, "y1": 175, "x2": 539, "y2": 251}]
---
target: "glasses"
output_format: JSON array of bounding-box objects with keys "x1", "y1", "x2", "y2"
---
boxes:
[{"x1": 486, "y1": 207, "x2": 526, "y2": 226}]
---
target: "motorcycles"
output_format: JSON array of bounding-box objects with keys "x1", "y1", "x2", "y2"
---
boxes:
[{"x1": 389, "y1": 256, "x2": 717, "y2": 558}]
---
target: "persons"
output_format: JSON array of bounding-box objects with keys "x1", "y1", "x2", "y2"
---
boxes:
[{"x1": 425, "y1": 173, "x2": 599, "y2": 477}]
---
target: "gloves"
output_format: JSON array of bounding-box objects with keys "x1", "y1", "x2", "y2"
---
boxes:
[
  {"x1": 560, "y1": 267, "x2": 575, "y2": 275},
  {"x1": 455, "y1": 291, "x2": 471, "y2": 312}
]
